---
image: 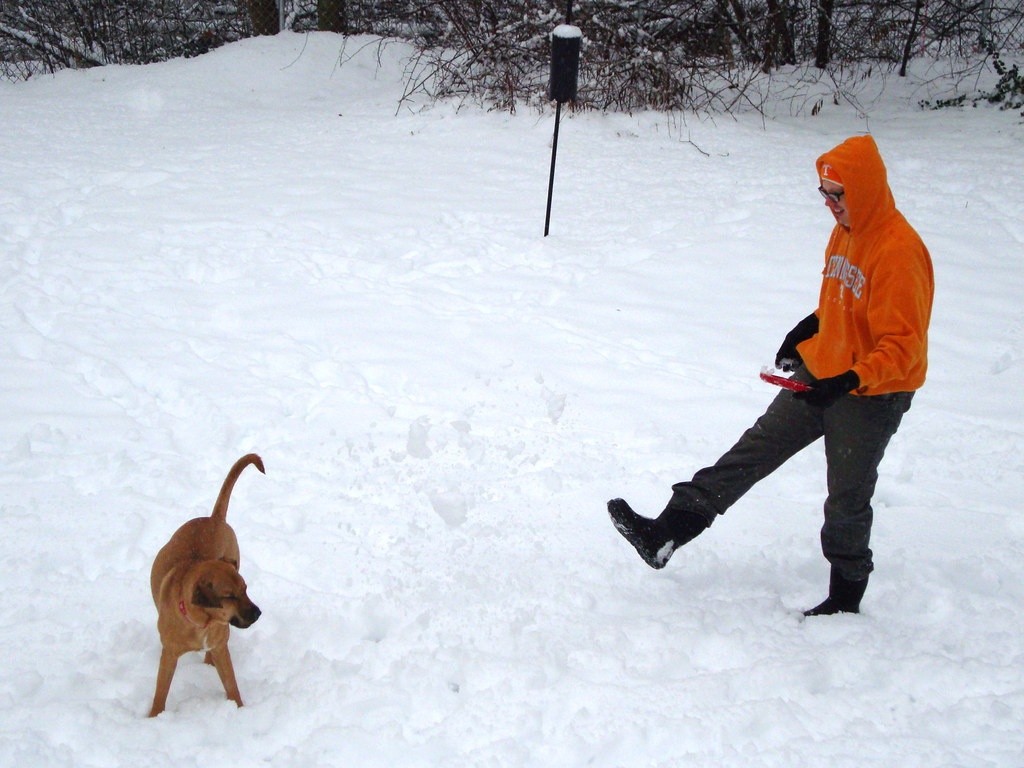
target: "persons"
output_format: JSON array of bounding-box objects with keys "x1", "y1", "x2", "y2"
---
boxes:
[{"x1": 606, "y1": 135, "x2": 934, "y2": 617}]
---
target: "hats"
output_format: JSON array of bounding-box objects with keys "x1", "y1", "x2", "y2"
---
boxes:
[{"x1": 821, "y1": 163, "x2": 843, "y2": 186}]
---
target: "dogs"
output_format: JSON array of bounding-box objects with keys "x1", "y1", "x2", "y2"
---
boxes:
[{"x1": 148, "y1": 454, "x2": 266, "y2": 718}]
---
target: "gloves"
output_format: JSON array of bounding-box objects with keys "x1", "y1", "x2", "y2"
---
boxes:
[
  {"x1": 792, "y1": 371, "x2": 859, "y2": 410},
  {"x1": 775, "y1": 313, "x2": 819, "y2": 372}
]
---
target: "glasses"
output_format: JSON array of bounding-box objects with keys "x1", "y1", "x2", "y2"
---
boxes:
[{"x1": 818, "y1": 187, "x2": 845, "y2": 202}]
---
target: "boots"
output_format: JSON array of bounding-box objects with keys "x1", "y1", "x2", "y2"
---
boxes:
[
  {"x1": 802, "y1": 565, "x2": 868, "y2": 616},
  {"x1": 607, "y1": 498, "x2": 707, "y2": 570}
]
